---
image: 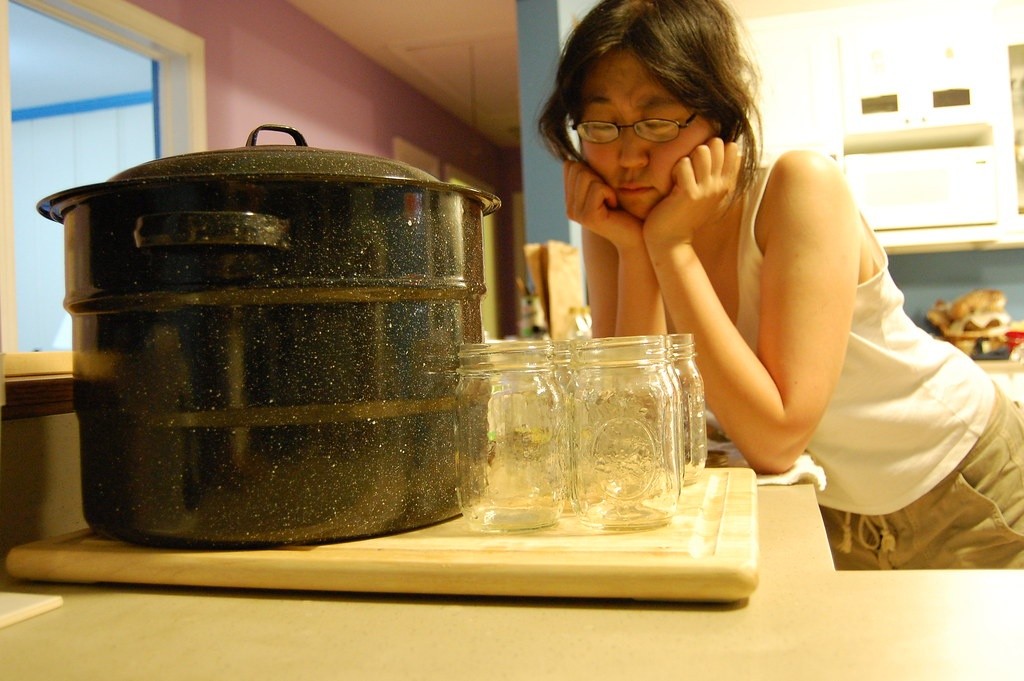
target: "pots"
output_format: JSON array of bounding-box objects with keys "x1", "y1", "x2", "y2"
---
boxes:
[{"x1": 39, "y1": 126, "x2": 502, "y2": 546}]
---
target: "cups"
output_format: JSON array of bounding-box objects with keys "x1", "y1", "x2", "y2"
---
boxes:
[
  {"x1": 572, "y1": 334, "x2": 677, "y2": 526},
  {"x1": 671, "y1": 332, "x2": 706, "y2": 485},
  {"x1": 456, "y1": 341, "x2": 564, "y2": 528},
  {"x1": 554, "y1": 339, "x2": 577, "y2": 514}
]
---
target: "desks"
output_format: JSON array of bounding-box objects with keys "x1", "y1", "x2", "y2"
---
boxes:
[{"x1": 0, "y1": 483, "x2": 1024, "y2": 681}]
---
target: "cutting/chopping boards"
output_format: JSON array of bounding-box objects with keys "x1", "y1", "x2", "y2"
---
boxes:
[{"x1": 8, "y1": 466, "x2": 756, "y2": 604}]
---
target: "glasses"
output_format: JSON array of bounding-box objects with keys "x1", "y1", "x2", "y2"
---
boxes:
[{"x1": 572, "y1": 111, "x2": 700, "y2": 144}]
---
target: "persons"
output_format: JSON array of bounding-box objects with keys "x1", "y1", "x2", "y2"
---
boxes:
[{"x1": 535, "y1": 0, "x2": 1023, "y2": 570}]
